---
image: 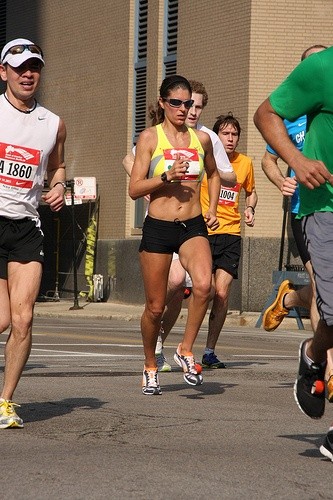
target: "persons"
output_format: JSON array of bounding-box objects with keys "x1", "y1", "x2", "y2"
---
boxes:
[
  {"x1": 128, "y1": 74, "x2": 221, "y2": 396},
  {"x1": 252, "y1": 46, "x2": 333, "y2": 460},
  {"x1": 122, "y1": 80, "x2": 236, "y2": 370},
  {"x1": 200, "y1": 112, "x2": 258, "y2": 368},
  {"x1": 0, "y1": 38, "x2": 66, "y2": 429},
  {"x1": 261, "y1": 44, "x2": 333, "y2": 402}
]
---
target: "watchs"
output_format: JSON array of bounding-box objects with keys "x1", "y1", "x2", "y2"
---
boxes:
[
  {"x1": 54, "y1": 182, "x2": 67, "y2": 193},
  {"x1": 161, "y1": 172, "x2": 171, "y2": 184}
]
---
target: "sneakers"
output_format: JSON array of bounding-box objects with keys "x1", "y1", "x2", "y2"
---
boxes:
[
  {"x1": 174, "y1": 342, "x2": 204, "y2": 386},
  {"x1": 153, "y1": 353, "x2": 173, "y2": 373},
  {"x1": 318, "y1": 429, "x2": 333, "y2": 462},
  {"x1": 201, "y1": 352, "x2": 225, "y2": 369},
  {"x1": 326, "y1": 375, "x2": 333, "y2": 401},
  {"x1": 0, "y1": 398, "x2": 24, "y2": 429},
  {"x1": 262, "y1": 280, "x2": 295, "y2": 332},
  {"x1": 293, "y1": 338, "x2": 328, "y2": 419},
  {"x1": 141, "y1": 365, "x2": 163, "y2": 395}
]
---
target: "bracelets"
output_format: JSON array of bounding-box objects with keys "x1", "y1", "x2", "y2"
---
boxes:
[{"x1": 248, "y1": 205, "x2": 255, "y2": 214}]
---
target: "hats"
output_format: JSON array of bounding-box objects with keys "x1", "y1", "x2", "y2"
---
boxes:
[{"x1": 0, "y1": 39, "x2": 46, "y2": 67}]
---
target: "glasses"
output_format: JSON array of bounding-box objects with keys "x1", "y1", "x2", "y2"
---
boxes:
[
  {"x1": 163, "y1": 97, "x2": 194, "y2": 109},
  {"x1": 2, "y1": 44, "x2": 45, "y2": 58}
]
